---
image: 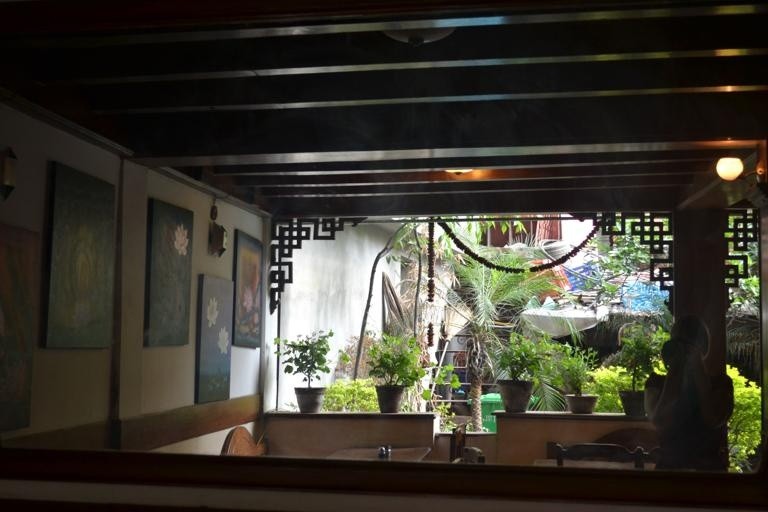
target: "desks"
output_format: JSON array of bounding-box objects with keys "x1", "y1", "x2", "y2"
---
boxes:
[
  {"x1": 532, "y1": 456, "x2": 657, "y2": 475},
  {"x1": 327, "y1": 445, "x2": 432, "y2": 466}
]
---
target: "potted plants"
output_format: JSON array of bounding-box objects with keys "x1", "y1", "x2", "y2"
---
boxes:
[
  {"x1": 495, "y1": 322, "x2": 664, "y2": 416},
  {"x1": 274, "y1": 321, "x2": 474, "y2": 431}
]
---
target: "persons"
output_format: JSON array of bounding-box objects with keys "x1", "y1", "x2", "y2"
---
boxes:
[{"x1": 643, "y1": 314, "x2": 733, "y2": 472}]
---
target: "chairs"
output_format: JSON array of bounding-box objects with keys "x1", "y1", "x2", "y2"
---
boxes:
[{"x1": 554, "y1": 443, "x2": 646, "y2": 471}]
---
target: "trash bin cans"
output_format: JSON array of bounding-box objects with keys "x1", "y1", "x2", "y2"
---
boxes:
[{"x1": 467, "y1": 393, "x2": 540, "y2": 432}]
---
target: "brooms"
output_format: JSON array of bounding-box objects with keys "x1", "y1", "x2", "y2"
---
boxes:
[{"x1": 430, "y1": 303, "x2": 473, "y2": 398}]
